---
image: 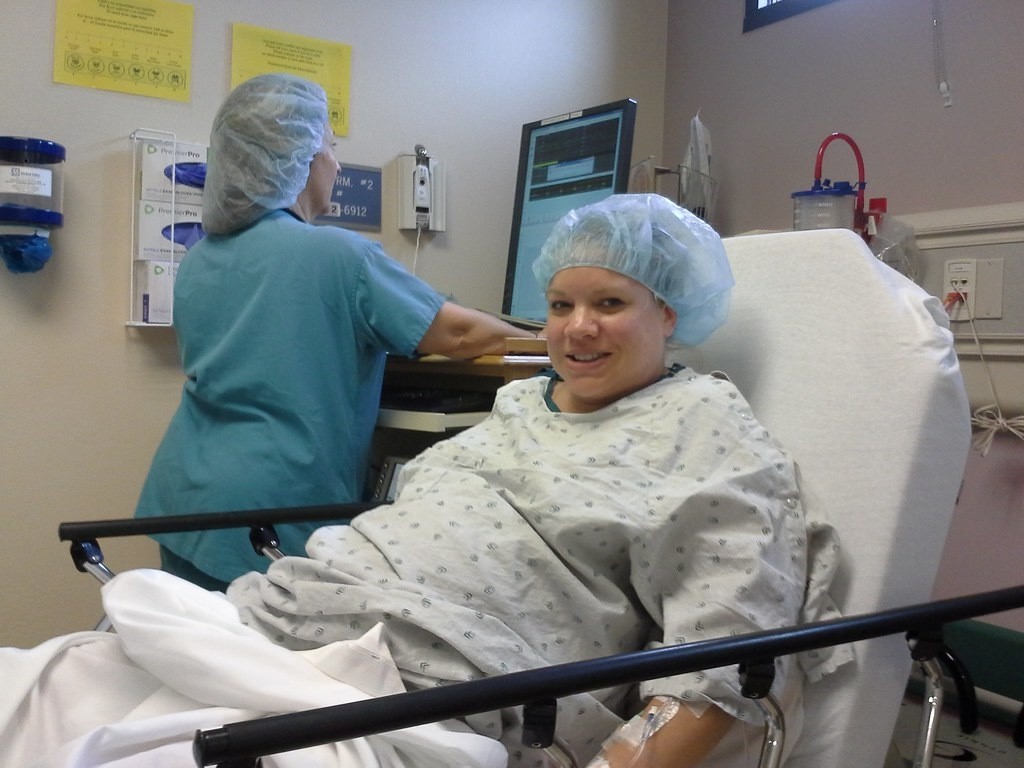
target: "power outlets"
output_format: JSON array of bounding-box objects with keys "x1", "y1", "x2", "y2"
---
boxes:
[{"x1": 942, "y1": 257, "x2": 975, "y2": 322}]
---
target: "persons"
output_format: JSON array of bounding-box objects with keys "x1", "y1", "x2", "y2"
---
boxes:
[
  {"x1": 225, "y1": 191, "x2": 809, "y2": 768},
  {"x1": 134, "y1": 75, "x2": 545, "y2": 595}
]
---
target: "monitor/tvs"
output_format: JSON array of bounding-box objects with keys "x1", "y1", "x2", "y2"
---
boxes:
[{"x1": 501, "y1": 99, "x2": 638, "y2": 331}]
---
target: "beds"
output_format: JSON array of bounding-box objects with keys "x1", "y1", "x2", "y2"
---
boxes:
[{"x1": 0, "y1": 229, "x2": 1024, "y2": 768}]
